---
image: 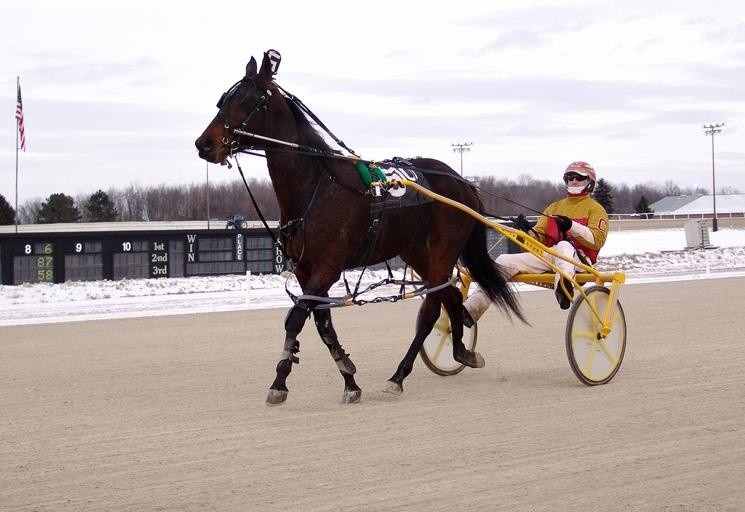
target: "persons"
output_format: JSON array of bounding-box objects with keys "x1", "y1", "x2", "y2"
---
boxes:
[{"x1": 462, "y1": 161, "x2": 608, "y2": 328}]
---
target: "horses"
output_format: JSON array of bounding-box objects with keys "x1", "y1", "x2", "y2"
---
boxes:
[{"x1": 191, "y1": 48, "x2": 532, "y2": 405}]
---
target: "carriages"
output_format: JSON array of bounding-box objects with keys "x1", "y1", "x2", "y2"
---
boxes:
[{"x1": 193, "y1": 49, "x2": 626, "y2": 407}]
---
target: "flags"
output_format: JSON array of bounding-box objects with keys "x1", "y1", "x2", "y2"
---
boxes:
[{"x1": 16, "y1": 88, "x2": 26, "y2": 152}]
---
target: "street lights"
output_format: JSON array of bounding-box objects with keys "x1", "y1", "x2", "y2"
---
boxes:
[
  {"x1": 451, "y1": 141, "x2": 474, "y2": 178},
  {"x1": 703, "y1": 122, "x2": 725, "y2": 232}
]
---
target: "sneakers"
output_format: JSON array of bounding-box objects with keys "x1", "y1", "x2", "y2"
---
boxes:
[
  {"x1": 462, "y1": 305, "x2": 474, "y2": 328},
  {"x1": 555, "y1": 278, "x2": 573, "y2": 309}
]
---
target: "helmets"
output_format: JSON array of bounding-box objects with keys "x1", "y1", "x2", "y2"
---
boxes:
[{"x1": 563, "y1": 162, "x2": 596, "y2": 196}]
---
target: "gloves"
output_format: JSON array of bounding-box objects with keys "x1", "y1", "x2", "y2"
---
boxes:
[
  {"x1": 513, "y1": 214, "x2": 529, "y2": 231},
  {"x1": 556, "y1": 215, "x2": 572, "y2": 231}
]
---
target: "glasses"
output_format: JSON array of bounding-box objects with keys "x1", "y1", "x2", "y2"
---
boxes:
[{"x1": 566, "y1": 174, "x2": 588, "y2": 181}]
